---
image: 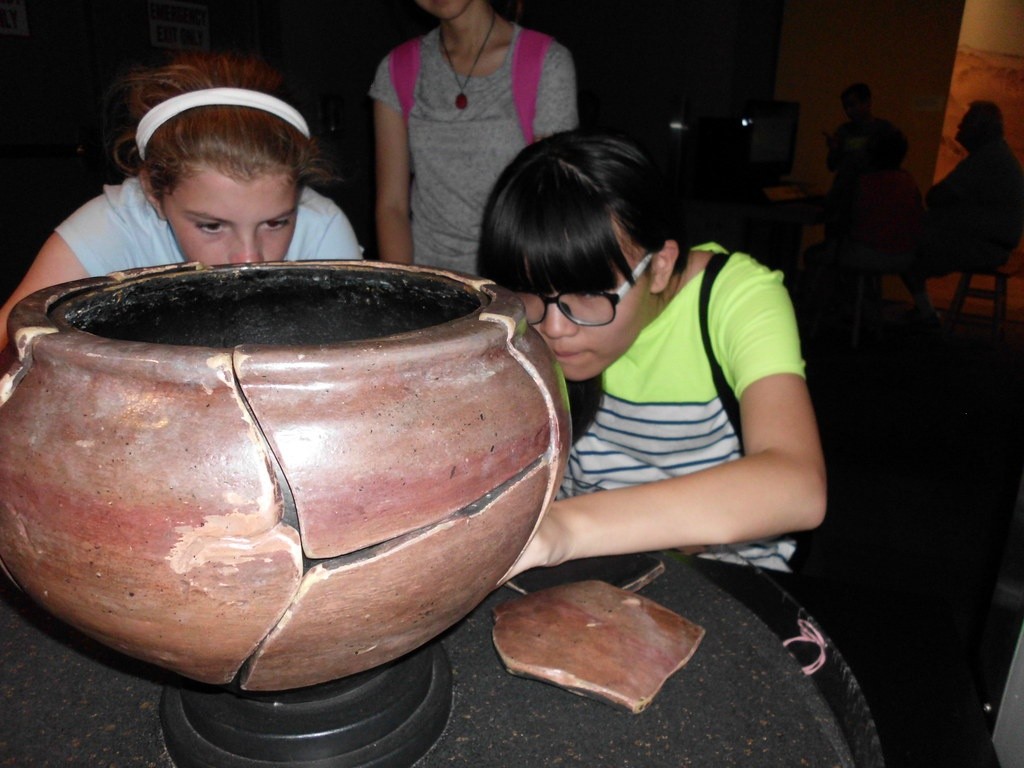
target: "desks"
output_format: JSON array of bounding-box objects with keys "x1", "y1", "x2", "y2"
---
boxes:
[{"x1": 0, "y1": 508, "x2": 892, "y2": 768}]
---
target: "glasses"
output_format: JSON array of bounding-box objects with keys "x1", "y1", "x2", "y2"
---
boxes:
[{"x1": 512, "y1": 250, "x2": 652, "y2": 326}]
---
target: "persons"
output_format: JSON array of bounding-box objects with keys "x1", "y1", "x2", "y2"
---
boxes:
[
  {"x1": 369, "y1": 0, "x2": 582, "y2": 278},
  {"x1": 802, "y1": 81, "x2": 1024, "y2": 372},
  {"x1": 475, "y1": 128, "x2": 828, "y2": 591},
  {"x1": 1, "y1": 51, "x2": 369, "y2": 355}
]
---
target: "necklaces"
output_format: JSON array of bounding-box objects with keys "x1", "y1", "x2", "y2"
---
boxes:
[{"x1": 439, "y1": 7, "x2": 497, "y2": 109}]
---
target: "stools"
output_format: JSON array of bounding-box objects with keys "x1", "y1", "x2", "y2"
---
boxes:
[{"x1": 950, "y1": 261, "x2": 1013, "y2": 323}]
